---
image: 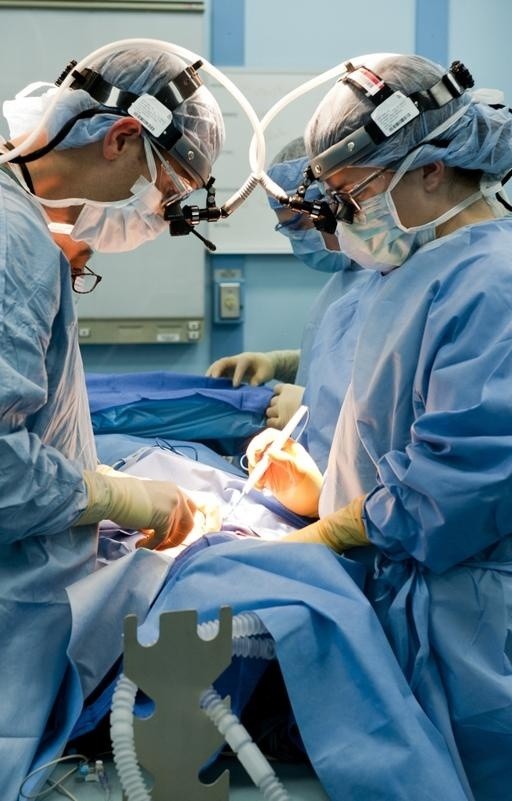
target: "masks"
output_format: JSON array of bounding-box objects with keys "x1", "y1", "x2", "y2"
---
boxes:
[
  {"x1": 334, "y1": 190, "x2": 418, "y2": 275},
  {"x1": 68, "y1": 183, "x2": 170, "y2": 255},
  {"x1": 282, "y1": 228, "x2": 352, "y2": 274},
  {"x1": 71, "y1": 273, "x2": 85, "y2": 306}
]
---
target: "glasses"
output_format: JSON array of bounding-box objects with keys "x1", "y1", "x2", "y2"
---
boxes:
[
  {"x1": 67, "y1": 263, "x2": 104, "y2": 295},
  {"x1": 274, "y1": 210, "x2": 311, "y2": 243},
  {"x1": 142, "y1": 130, "x2": 194, "y2": 210},
  {"x1": 327, "y1": 138, "x2": 448, "y2": 226}
]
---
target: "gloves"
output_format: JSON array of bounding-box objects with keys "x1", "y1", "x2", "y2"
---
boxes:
[
  {"x1": 265, "y1": 381, "x2": 305, "y2": 429},
  {"x1": 205, "y1": 348, "x2": 303, "y2": 390},
  {"x1": 244, "y1": 426, "x2": 324, "y2": 518},
  {"x1": 73, "y1": 468, "x2": 198, "y2": 552},
  {"x1": 275, "y1": 494, "x2": 370, "y2": 548}
]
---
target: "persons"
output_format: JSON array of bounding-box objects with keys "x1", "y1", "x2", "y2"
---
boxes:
[
  {"x1": 208, "y1": 134, "x2": 387, "y2": 433},
  {"x1": 0, "y1": 39, "x2": 225, "y2": 801},
  {"x1": 247, "y1": 54, "x2": 512, "y2": 801}
]
